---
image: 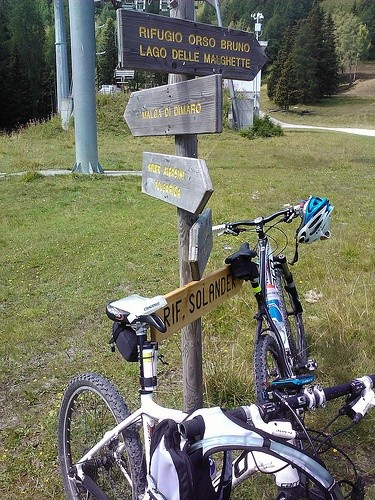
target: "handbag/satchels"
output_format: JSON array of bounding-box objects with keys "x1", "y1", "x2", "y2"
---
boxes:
[{"x1": 108, "y1": 321, "x2": 139, "y2": 363}]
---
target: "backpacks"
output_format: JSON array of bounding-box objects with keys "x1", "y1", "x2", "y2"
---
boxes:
[{"x1": 144, "y1": 407, "x2": 346, "y2": 500}]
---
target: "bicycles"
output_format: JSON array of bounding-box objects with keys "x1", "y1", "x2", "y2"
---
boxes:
[
  {"x1": 58, "y1": 293, "x2": 375, "y2": 500},
  {"x1": 212, "y1": 197, "x2": 317, "y2": 432}
]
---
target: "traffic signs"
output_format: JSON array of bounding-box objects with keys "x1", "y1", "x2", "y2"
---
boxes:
[{"x1": 114, "y1": 7, "x2": 267, "y2": 342}]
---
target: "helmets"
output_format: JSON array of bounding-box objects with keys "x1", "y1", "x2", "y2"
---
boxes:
[{"x1": 295, "y1": 194, "x2": 335, "y2": 244}]
